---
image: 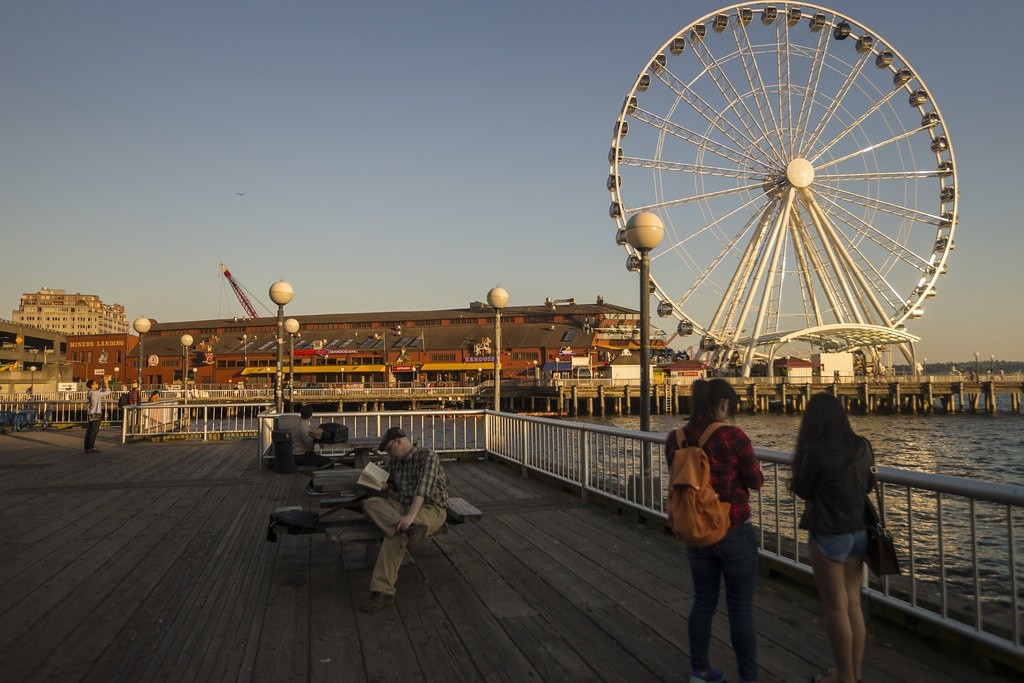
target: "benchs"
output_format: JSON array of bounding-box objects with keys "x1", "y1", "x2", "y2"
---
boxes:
[{"x1": 275, "y1": 454, "x2": 469, "y2": 542}]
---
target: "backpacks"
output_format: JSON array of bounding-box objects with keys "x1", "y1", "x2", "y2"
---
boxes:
[{"x1": 667, "y1": 421, "x2": 732, "y2": 546}]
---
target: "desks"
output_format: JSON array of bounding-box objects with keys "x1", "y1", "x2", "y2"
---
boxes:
[
  {"x1": 342, "y1": 438, "x2": 380, "y2": 468},
  {"x1": 309, "y1": 469, "x2": 370, "y2": 491}
]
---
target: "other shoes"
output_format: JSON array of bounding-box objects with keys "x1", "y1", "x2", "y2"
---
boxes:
[
  {"x1": 306, "y1": 486, "x2": 329, "y2": 496},
  {"x1": 690, "y1": 667, "x2": 724, "y2": 683},
  {"x1": 407, "y1": 524, "x2": 428, "y2": 549},
  {"x1": 361, "y1": 592, "x2": 394, "y2": 612},
  {"x1": 84, "y1": 447, "x2": 99, "y2": 453}
]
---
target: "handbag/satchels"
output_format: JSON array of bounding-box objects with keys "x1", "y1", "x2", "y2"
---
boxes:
[
  {"x1": 88, "y1": 413, "x2": 100, "y2": 422},
  {"x1": 863, "y1": 521, "x2": 901, "y2": 577},
  {"x1": 266, "y1": 510, "x2": 319, "y2": 544},
  {"x1": 118, "y1": 393, "x2": 130, "y2": 408}
]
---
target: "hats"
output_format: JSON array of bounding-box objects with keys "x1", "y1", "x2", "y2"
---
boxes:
[
  {"x1": 378, "y1": 426, "x2": 406, "y2": 452},
  {"x1": 133, "y1": 382, "x2": 138, "y2": 389}
]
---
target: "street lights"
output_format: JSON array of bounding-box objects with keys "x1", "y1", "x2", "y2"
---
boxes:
[
  {"x1": 923, "y1": 357, "x2": 927, "y2": 376},
  {"x1": 30, "y1": 365, "x2": 36, "y2": 402},
  {"x1": 242, "y1": 334, "x2": 247, "y2": 367},
  {"x1": 114, "y1": 367, "x2": 119, "y2": 391},
  {"x1": 134, "y1": 317, "x2": 152, "y2": 433},
  {"x1": 269, "y1": 280, "x2": 294, "y2": 415},
  {"x1": 487, "y1": 287, "x2": 509, "y2": 449},
  {"x1": 990, "y1": 354, "x2": 995, "y2": 380},
  {"x1": 180, "y1": 334, "x2": 194, "y2": 426},
  {"x1": 974, "y1": 351, "x2": 980, "y2": 383},
  {"x1": 283, "y1": 317, "x2": 301, "y2": 413},
  {"x1": 193, "y1": 367, "x2": 198, "y2": 389},
  {"x1": 623, "y1": 210, "x2": 665, "y2": 475}
]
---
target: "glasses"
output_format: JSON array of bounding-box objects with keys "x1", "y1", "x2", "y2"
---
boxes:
[{"x1": 386, "y1": 440, "x2": 397, "y2": 454}]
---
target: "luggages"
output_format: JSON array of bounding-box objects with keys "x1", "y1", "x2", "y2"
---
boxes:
[{"x1": 316, "y1": 423, "x2": 348, "y2": 444}]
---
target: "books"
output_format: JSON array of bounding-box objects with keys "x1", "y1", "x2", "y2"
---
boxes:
[
  {"x1": 308, "y1": 426, "x2": 323, "y2": 439},
  {"x1": 357, "y1": 462, "x2": 390, "y2": 491}
]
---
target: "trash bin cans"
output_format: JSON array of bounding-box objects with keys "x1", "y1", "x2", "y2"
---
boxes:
[{"x1": 272, "y1": 430, "x2": 293, "y2": 474}]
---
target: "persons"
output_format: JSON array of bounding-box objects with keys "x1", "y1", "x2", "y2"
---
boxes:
[
  {"x1": 651, "y1": 349, "x2": 690, "y2": 364},
  {"x1": 148, "y1": 390, "x2": 160, "y2": 403},
  {"x1": 84, "y1": 376, "x2": 111, "y2": 453},
  {"x1": 665, "y1": 379, "x2": 764, "y2": 683},
  {"x1": 218, "y1": 384, "x2": 248, "y2": 397},
  {"x1": 292, "y1": 406, "x2": 335, "y2": 496},
  {"x1": 833, "y1": 368, "x2": 1005, "y2": 382},
  {"x1": 128, "y1": 383, "x2": 142, "y2": 430},
  {"x1": 274, "y1": 373, "x2": 292, "y2": 413},
  {"x1": 787, "y1": 393, "x2": 878, "y2": 683},
  {"x1": 362, "y1": 427, "x2": 448, "y2": 612},
  {"x1": 284, "y1": 380, "x2": 447, "y2": 394}
]
